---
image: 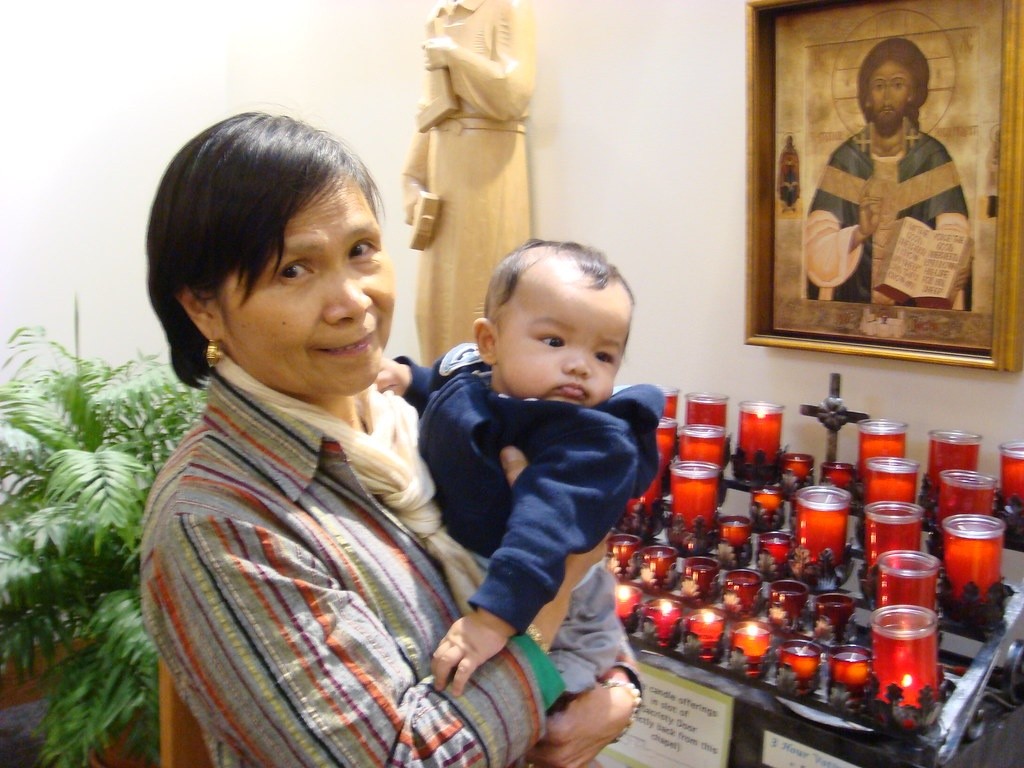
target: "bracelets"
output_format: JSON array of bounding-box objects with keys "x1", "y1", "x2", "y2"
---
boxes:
[
  {"x1": 613, "y1": 662, "x2": 643, "y2": 713},
  {"x1": 599, "y1": 679, "x2": 643, "y2": 743},
  {"x1": 528, "y1": 625, "x2": 548, "y2": 653}
]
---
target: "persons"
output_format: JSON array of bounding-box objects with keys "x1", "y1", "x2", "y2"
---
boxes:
[
  {"x1": 373, "y1": 239, "x2": 667, "y2": 768},
  {"x1": 399, "y1": 0, "x2": 536, "y2": 367},
  {"x1": 141, "y1": 113, "x2": 644, "y2": 768}
]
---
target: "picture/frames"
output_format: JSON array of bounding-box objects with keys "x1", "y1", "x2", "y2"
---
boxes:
[{"x1": 745, "y1": 0, "x2": 1024, "y2": 372}]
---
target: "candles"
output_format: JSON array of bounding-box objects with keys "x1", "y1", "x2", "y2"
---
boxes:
[
  {"x1": 922, "y1": 428, "x2": 982, "y2": 507},
  {"x1": 941, "y1": 514, "x2": 1005, "y2": 606},
  {"x1": 877, "y1": 551, "x2": 940, "y2": 613},
  {"x1": 601, "y1": 385, "x2": 872, "y2": 702},
  {"x1": 933, "y1": 469, "x2": 997, "y2": 536},
  {"x1": 999, "y1": 440, "x2": 1024, "y2": 514},
  {"x1": 865, "y1": 455, "x2": 920, "y2": 504},
  {"x1": 857, "y1": 419, "x2": 907, "y2": 483},
  {"x1": 864, "y1": 501, "x2": 925, "y2": 602},
  {"x1": 871, "y1": 604, "x2": 937, "y2": 730}
]
